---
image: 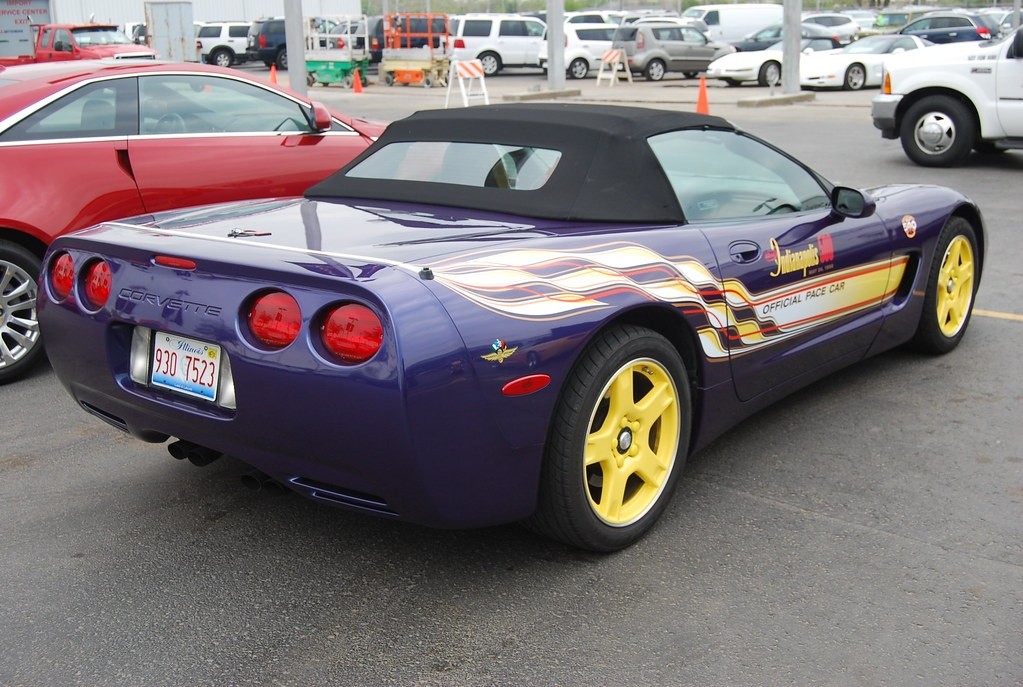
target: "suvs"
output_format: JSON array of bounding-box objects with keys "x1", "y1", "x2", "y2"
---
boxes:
[{"x1": 36, "y1": 9, "x2": 737, "y2": 86}]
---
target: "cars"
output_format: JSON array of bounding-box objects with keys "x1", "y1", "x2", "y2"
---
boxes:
[
  {"x1": 0, "y1": 59, "x2": 389, "y2": 385},
  {"x1": 37, "y1": 102, "x2": 989, "y2": 553},
  {"x1": 708, "y1": 6, "x2": 1023, "y2": 168}
]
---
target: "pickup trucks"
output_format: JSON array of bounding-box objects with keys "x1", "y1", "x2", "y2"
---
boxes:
[{"x1": 0, "y1": 25, "x2": 154, "y2": 66}]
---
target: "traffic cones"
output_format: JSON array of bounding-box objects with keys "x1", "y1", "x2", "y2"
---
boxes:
[
  {"x1": 268, "y1": 63, "x2": 277, "y2": 84},
  {"x1": 353, "y1": 69, "x2": 363, "y2": 93},
  {"x1": 694, "y1": 75, "x2": 710, "y2": 116}
]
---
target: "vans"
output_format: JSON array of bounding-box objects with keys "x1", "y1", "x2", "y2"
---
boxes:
[
  {"x1": 451, "y1": 13, "x2": 547, "y2": 77},
  {"x1": 684, "y1": 5, "x2": 787, "y2": 47}
]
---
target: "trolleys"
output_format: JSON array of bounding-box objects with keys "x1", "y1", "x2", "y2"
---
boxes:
[
  {"x1": 302, "y1": 13, "x2": 370, "y2": 88},
  {"x1": 381, "y1": 12, "x2": 450, "y2": 88}
]
